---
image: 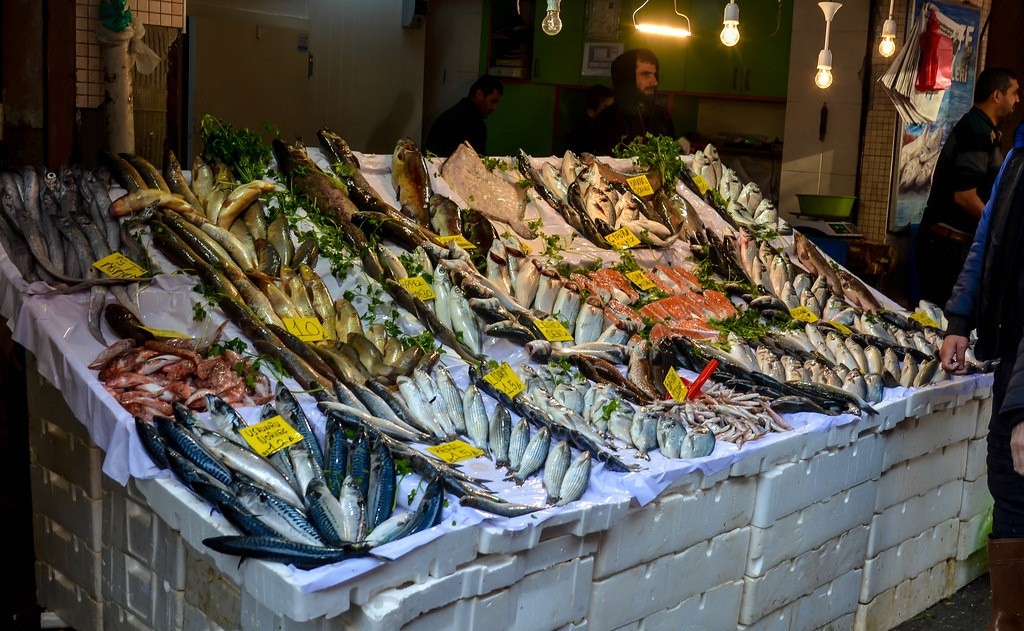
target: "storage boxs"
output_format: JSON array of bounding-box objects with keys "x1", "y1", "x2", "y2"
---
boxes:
[{"x1": 25, "y1": 349, "x2": 995, "y2": 631}]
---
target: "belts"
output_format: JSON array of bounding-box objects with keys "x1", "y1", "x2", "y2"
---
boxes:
[{"x1": 929, "y1": 221, "x2": 971, "y2": 243}]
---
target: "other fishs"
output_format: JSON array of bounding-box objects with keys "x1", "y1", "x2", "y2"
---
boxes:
[{"x1": 1, "y1": 125, "x2": 1002, "y2": 571}]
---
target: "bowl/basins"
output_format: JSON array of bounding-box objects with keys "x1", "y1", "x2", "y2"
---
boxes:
[{"x1": 795, "y1": 194, "x2": 857, "y2": 217}]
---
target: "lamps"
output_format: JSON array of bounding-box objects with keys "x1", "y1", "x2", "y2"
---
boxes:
[{"x1": 633, "y1": 0, "x2": 693, "y2": 39}]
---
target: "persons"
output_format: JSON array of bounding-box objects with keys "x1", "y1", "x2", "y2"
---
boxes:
[
  {"x1": 428, "y1": 75, "x2": 504, "y2": 157},
  {"x1": 915, "y1": 67, "x2": 1021, "y2": 309},
  {"x1": 589, "y1": 48, "x2": 675, "y2": 158},
  {"x1": 571, "y1": 85, "x2": 616, "y2": 144},
  {"x1": 940, "y1": 133, "x2": 1024, "y2": 631},
  {"x1": 951, "y1": 31, "x2": 960, "y2": 55}
]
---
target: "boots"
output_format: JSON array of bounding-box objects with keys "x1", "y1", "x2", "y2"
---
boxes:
[{"x1": 986, "y1": 534, "x2": 1024, "y2": 631}]
